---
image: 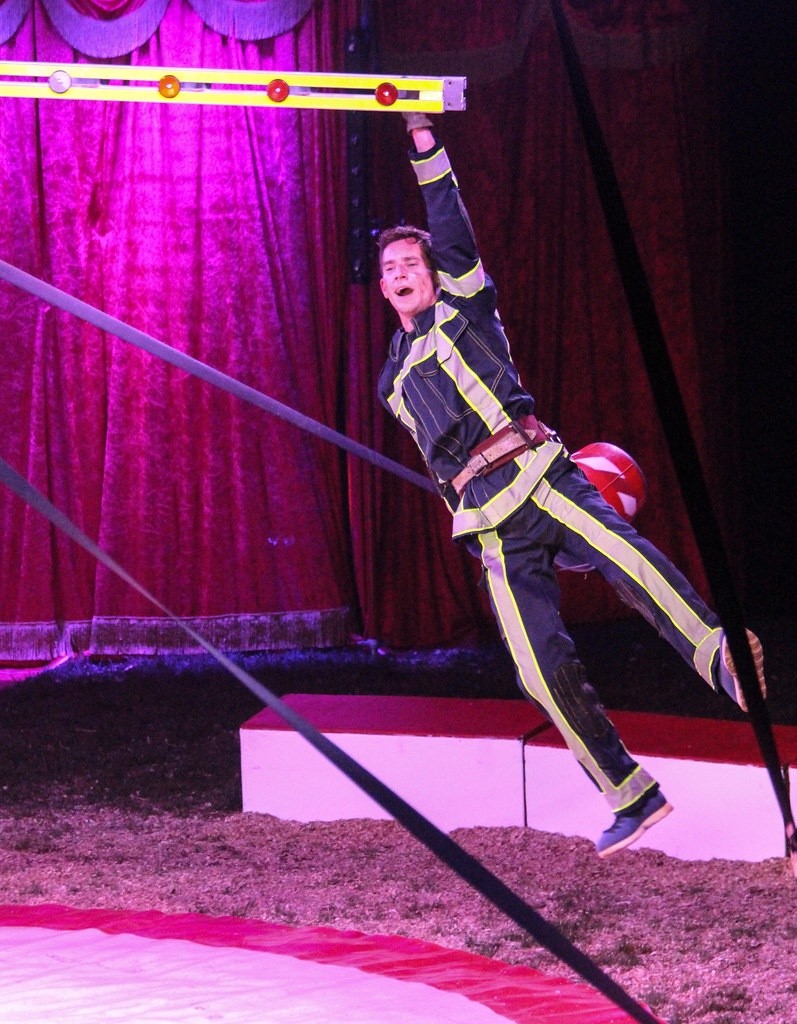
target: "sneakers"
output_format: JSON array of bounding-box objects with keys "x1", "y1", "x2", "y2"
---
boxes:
[
  {"x1": 597, "y1": 789, "x2": 673, "y2": 859},
  {"x1": 716, "y1": 626, "x2": 767, "y2": 713}
]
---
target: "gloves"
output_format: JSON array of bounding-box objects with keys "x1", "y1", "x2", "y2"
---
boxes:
[{"x1": 396, "y1": 76, "x2": 434, "y2": 136}]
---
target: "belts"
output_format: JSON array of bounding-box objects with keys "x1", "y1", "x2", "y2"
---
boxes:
[{"x1": 451, "y1": 429, "x2": 538, "y2": 495}]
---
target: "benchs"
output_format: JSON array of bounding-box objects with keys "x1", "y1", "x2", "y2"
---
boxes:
[
  {"x1": 524, "y1": 708, "x2": 797, "y2": 862},
  {"x1": 235, "y1": 690, "x2": 551, "y2": 835}
]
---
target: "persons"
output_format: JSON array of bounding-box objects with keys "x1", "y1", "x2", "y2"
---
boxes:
[{"x1": 376, "y1": 75, "x2": 766, "y2": 858}]
